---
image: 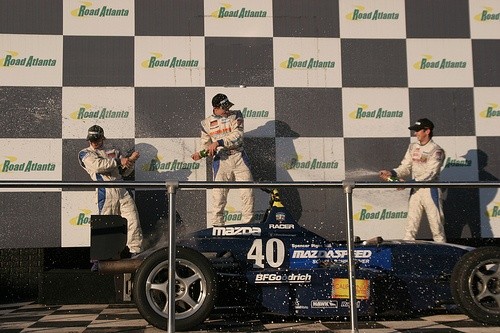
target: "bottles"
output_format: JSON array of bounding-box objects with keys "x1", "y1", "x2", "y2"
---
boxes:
[
  {"x1": 193, "y1": 150, "x2": 216, "y2": 159},
  {"x1": 379, "y1": 171, "x2": 400, "y2": 183}
]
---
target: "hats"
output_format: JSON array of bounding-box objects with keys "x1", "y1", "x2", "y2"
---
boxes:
[
  {"x1": 408, "y1": 118, "x2": 433, "y2": 132},
  {"x1": 211, "y1": 93, "x2": 234, "y2": 111},
  {"x1": 86, "y1": 125, "x2": 106, "y2": 142}
]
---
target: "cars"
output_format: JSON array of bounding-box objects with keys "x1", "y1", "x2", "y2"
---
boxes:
[{"x1": 130, "y1": 186, "x2": 500, "y2": 330}]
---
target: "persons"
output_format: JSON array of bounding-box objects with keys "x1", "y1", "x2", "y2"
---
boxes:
[
  {"x1": 378, "y1": 119, "x2": 447, "y2": 242},
  {"x1": 78, "y1": 125, "x2": 144, "y2": 271},
  {"x1": 192, "y1": 93, "x2": 254, "y2": 226}
]
